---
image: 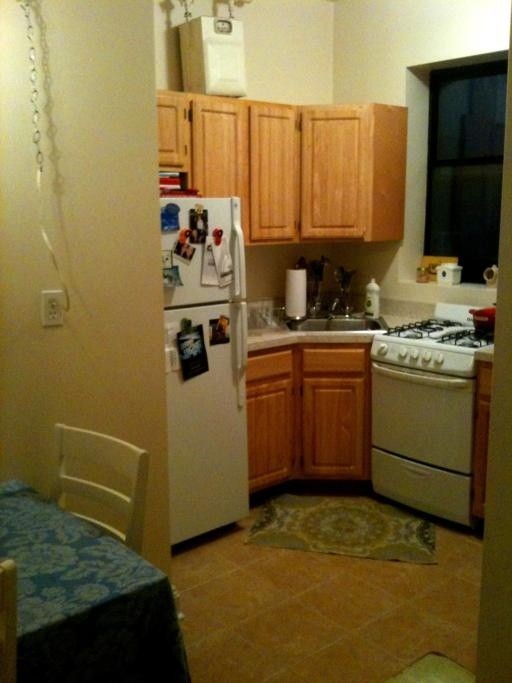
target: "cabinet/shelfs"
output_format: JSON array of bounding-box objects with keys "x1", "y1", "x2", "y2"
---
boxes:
[{"x1": 244, "y1": 350, "x2": 370, "y2": 493}]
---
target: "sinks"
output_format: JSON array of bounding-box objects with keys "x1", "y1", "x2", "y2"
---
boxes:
[{"x1": 286, "y1": 315, "x2": 390, "y2": 335}]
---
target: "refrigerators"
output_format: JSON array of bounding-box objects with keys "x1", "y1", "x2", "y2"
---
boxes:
[{"x1": 160, "y1": 196, "x2": 249, "y2": 546}]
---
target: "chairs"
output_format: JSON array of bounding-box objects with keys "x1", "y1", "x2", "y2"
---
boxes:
[{"x1": 55, "y1": 421, "x2": 149, "y2": 553}]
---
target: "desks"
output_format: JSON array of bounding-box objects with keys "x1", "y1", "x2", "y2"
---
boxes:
[{"x1": 0, "y1": 479, "x2": 167, "y2": 642}]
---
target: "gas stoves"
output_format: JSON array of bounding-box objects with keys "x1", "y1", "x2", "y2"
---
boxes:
[{"x1": 370, "y1": 303, "x2": 494, "y2": 378}]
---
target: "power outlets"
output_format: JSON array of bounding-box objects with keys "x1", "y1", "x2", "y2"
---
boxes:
[{"x1": 41, "y1": 290, "x2": 62, "y2": 325}]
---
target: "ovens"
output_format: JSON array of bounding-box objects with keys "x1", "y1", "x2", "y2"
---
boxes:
[{"x1": 371, "y1": 360, "x2": 477, "y2": 526}]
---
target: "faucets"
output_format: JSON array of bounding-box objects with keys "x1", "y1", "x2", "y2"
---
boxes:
[{"x1": 326, "y1": 311, "x2": 336, "y2": 323}]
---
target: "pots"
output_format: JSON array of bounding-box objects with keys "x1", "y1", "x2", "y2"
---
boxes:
[{"x1": 469, "y1": 302, "x2": 496, "y2": 341}]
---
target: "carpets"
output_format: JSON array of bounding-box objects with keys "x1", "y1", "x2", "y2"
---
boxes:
[{"x1": 241, "y1": 493, "x2": 438, "y2": 566}]
